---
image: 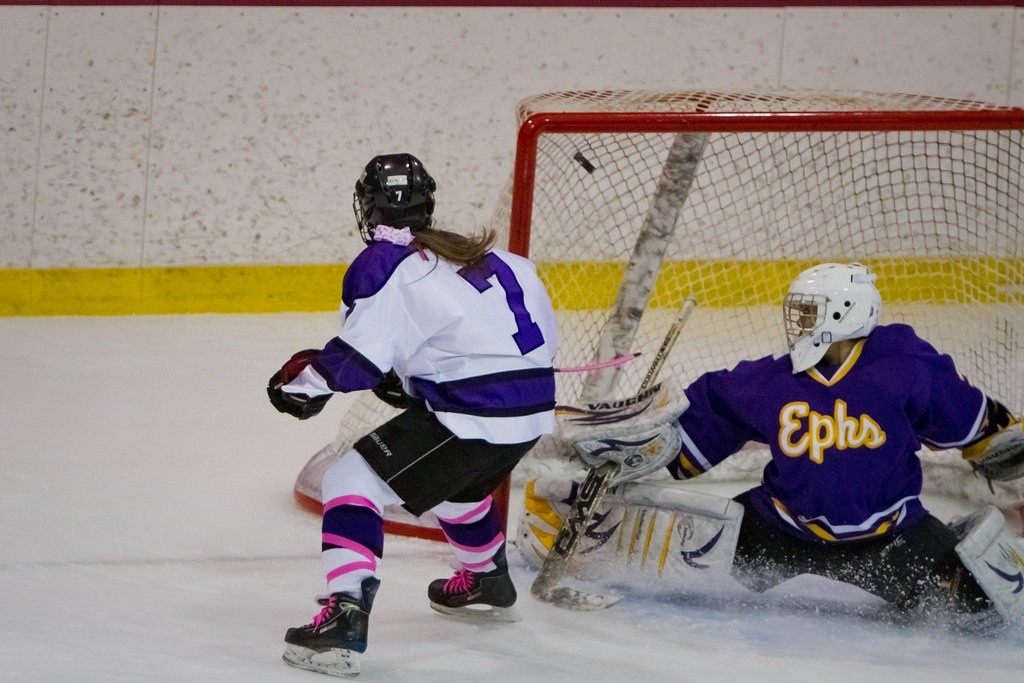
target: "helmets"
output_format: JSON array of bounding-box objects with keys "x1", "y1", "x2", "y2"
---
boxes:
[
  {"x1": 355, "y1": 154, "x2": 437, "y2": 228},
  {"x1": 787, "y1": 261, "x2": 883, "y2": 374}
]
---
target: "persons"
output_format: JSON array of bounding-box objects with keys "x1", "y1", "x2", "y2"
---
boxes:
[
  {"x1": 262, "y1": 151, "x2": 562, "y2": 656},
  {"x1": 514, "y1": 260, "x2": 1024, "y2": 639}
]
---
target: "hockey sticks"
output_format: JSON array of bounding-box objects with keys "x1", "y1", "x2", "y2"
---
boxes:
[
  {"x1": 280, "y1": 351, "x2": 643, "y2": 394},
  {"x1": 528, "y1": 297, "x2": 698, "y2": 612}
]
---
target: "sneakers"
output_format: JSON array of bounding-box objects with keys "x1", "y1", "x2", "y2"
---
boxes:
[
  {"x1": 282, "y1": 577, "x2": 380, "y2": 677},
  {"x1": 428, "y1": 544, "x2": 522, "y2": 622}
]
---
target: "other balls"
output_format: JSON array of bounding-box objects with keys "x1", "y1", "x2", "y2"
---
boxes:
[{"x1": 573, "y1": 152, "x2": 596, "y2": 174}]
